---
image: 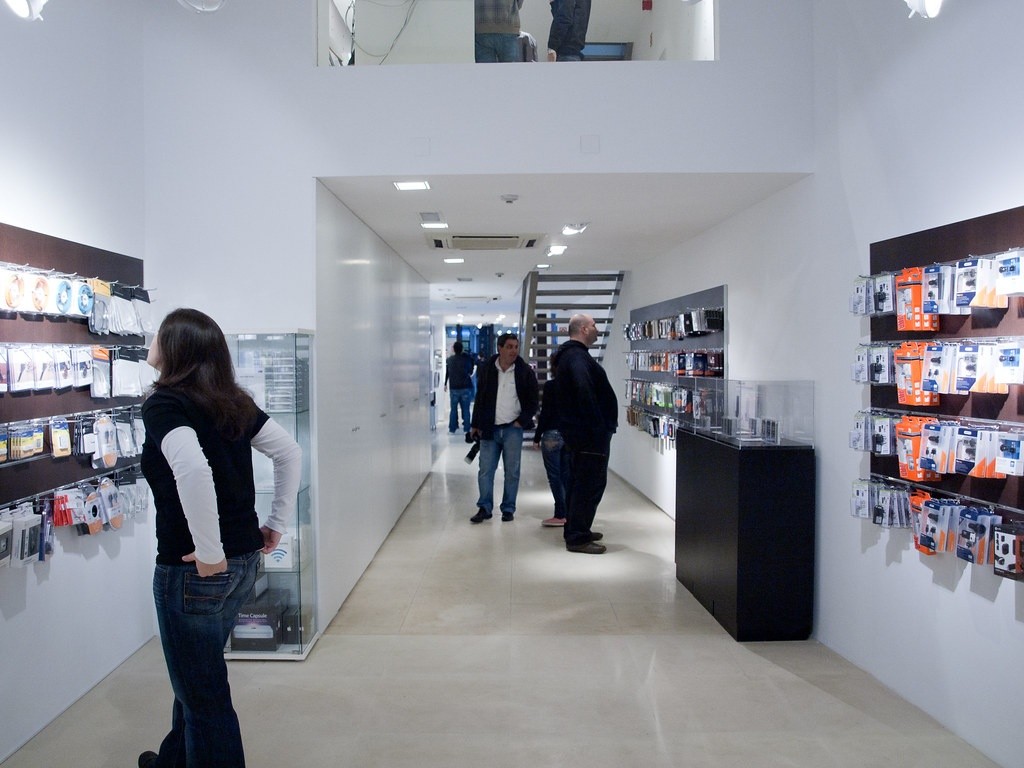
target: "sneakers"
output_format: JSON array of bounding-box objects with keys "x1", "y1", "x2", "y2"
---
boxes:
[{"x1": 542, "y1": 517, "x2": 566, "y2": 525}]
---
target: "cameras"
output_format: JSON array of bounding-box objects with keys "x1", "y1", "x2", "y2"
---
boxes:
[{"x1": 464, "y1": 430, "x2": 493, "y2": 465}]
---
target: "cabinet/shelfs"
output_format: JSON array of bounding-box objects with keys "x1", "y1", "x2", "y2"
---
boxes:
[{"x1": 223, "y1": 334, "x2": 318, "y2": 661}]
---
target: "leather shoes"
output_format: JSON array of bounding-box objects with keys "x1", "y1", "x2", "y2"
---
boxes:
[
  {"x1": 586, "y1": 532, "x2": 603, "y2": 541},
  {"x1": 567, "y1": 542, "x2": 607, "y2": 554}
]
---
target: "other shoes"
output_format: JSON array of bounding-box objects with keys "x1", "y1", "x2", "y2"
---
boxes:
[
  {"x1": 138, "y1": 751, "x2": 158, "y2": 768},
  {"x1": 502, "y1": 510, "x2": 513, "y2": 521},
  {"x1": 448, "y1": 431, "x2": 455, "y2": 434},
  {"x1": 470, "y1": 506, "x2": 492, "y2": 522},
  {"x1": 464, "y1": 432, "x2": 467, "y2": 434}
]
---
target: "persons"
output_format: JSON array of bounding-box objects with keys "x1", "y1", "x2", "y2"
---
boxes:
[
  {"x1": 462, "y1": 349, "x2": 484, "y2": 378},
  {"x1": 534, "y1": 314, "x2": 618, "y2": 555},
  {"x1": 470, "y1": 334, "x2": 538, "y2": 523},
  {"x1": 137, "y1": 308, "x2": 304, "y2": 768},
  {"x1": 475, "y1": 0, "x2": 591, "y2": 63},
  {"x1": 443, "y1": 342, "x2": 473, "y2": 434}
]
